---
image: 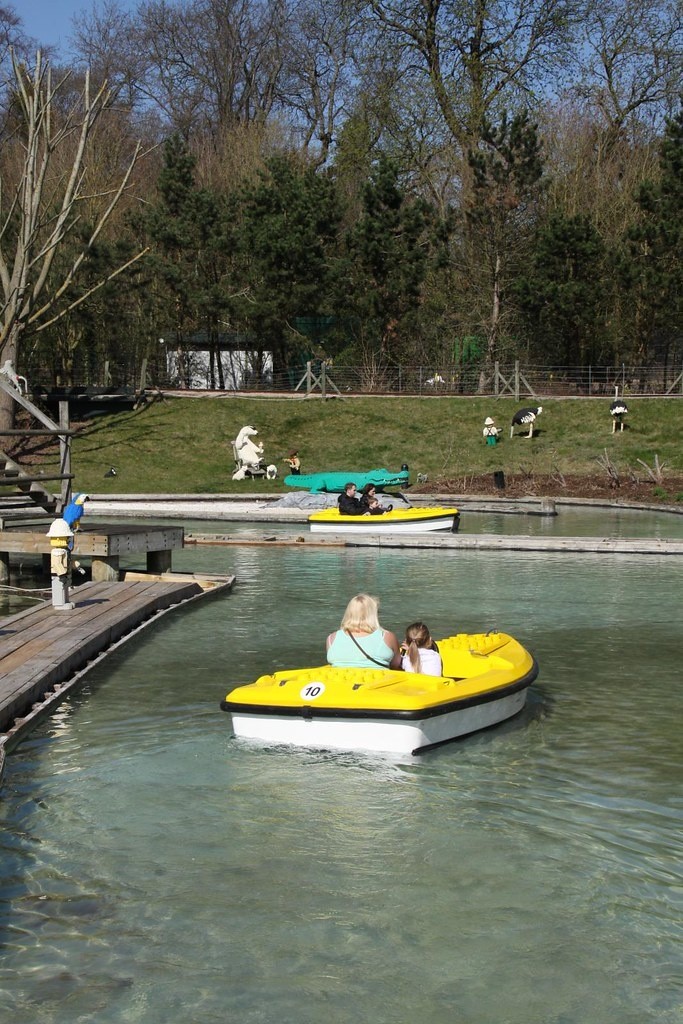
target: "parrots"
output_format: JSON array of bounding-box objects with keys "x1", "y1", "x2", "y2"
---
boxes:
[{"x1": 63, "y1": 494, "x2": 90, "y2": 551}]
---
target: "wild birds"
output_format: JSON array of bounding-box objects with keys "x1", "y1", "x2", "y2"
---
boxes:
[{"x1": 417, "y1": 473, "x2": 428, "y2": 484}]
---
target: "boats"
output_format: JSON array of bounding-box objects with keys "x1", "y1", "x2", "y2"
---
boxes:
[
  {"x1": 219, "y1": 630, "x2": 541, "y2": 757},
  {"x1": 307, "y1": 504, "x2": 460, "y2": 534}
]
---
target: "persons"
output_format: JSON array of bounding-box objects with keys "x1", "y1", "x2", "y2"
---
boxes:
[
  {"x1": 324, "y1": 594, "x2": 409, "y2": 670},
  {"x1": 360, "y1": 484, "x2": 375, "y2": 509},
  {"x1": 482, "y1": 417, "x2": 499, "y2": 447},
  {"x1": 362, "y1": 499, "x2": 385, "y2": 515},
  {"x1": 337, "y1": 483, "x2": 366, "y2": 515},
  {"x1": 281, "y1": 448, "x2": 301, "y2": 475},
  {"x1": 402, "y1": 623, "x2": 442, "y2": 677}
]
